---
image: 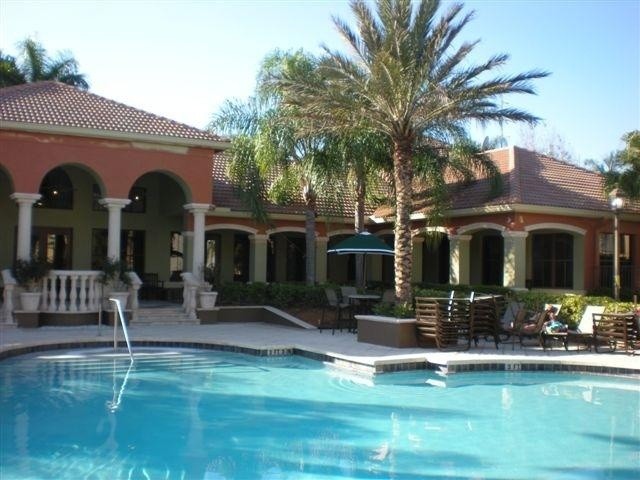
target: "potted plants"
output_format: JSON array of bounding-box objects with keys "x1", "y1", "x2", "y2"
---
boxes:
[
  {"x1": 11, "y1": 255, "x2": 52, "y2": 312},
  {"x1": 94, "y1": 256, "x2": 132, "y2": 311}
]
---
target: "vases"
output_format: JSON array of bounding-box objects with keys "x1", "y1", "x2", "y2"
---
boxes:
[{"x1": 199, "y1": 291, "x2": 218, "y2": 308}]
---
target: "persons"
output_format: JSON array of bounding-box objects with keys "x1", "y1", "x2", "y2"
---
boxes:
[{"x1": 501, "y1": 303, "x2": 555, "y2": 333}]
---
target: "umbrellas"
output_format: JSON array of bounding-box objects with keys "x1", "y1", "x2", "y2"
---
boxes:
[{"x1": 325, "y1": 228, "x2": 395, "y2": 295}]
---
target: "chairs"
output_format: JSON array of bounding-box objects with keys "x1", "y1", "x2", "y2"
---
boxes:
[
  {"x1": 414, "y1": 291, "x2": 640, "y2": 356},
  {"x1": 320, "y1": 286, "x2": 394, "y2": 335}
]
